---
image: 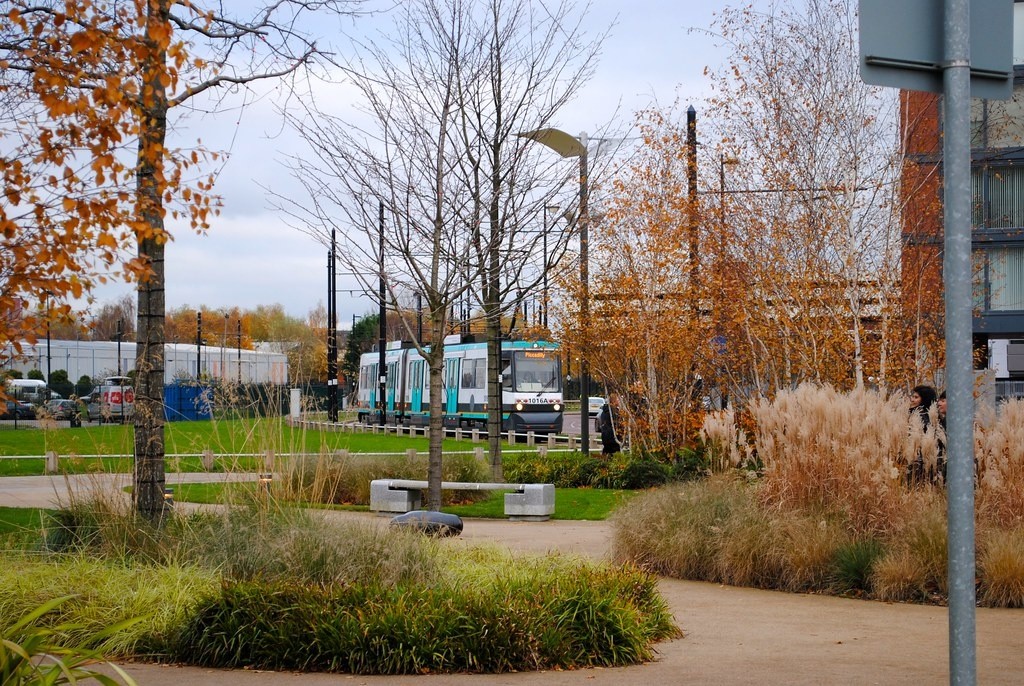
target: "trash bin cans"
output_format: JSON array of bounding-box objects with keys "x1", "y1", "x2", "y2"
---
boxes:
[{"x1": 163, "y1": 384, "x2": 214, "y2": 421}]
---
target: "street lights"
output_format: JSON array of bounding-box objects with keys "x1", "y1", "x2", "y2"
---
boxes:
[
  {"x1": 219, "y1": 312, "x2": 230, "y2": 381},
  {"x1": 685, "y1": 140, "x2": 743, "y2": 410},
  {"x1": 45, "y1": 289, "x2": 55, "y2": 400},
  {"x1": 542, "y1": 199, "x2": 560, "y2": 330},
  {"x1": 515, "y1": 129, "x2": 662, "y2": 462},
  {"x1": 350, "y1": 314, "x2": 362, "y2": 395}
]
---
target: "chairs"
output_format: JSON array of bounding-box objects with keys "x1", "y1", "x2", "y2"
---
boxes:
[{"x1": 522, "y1": 371, "x2": 539, "y2": 383}]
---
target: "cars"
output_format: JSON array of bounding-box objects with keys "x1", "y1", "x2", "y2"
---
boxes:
[
  {"x1": 0, "y1": 374, "x2": 135, "y2": 419},
  {"x1": 588, "y1": 396, "x2": 606, "y2": 415}
]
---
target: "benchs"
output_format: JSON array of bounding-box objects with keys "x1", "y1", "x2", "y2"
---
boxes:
[{"x1": 370, "y1": 478, "x2": 555, "y2": 522}]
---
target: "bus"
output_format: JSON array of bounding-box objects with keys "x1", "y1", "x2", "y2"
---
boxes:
[{"x1": 352, "y1": 337, "x2": 565, "y2": 440}]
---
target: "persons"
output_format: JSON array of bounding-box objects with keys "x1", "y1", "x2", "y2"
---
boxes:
[
  {"x1": 936, "y1": 390, "x2": 946, "y2": 487},
  {"x1": 908, "y1": 383, "x2": 936, "y2": 485},
  {"x1": 463, "y1": 373, "x2": 472, "y2": 386},
  {"x1": 522, "y1": 371, "x2": 539, "y2": 383},
  {"x1": 600, "y1": 393, "x2": 621, "y2": 461}
]
